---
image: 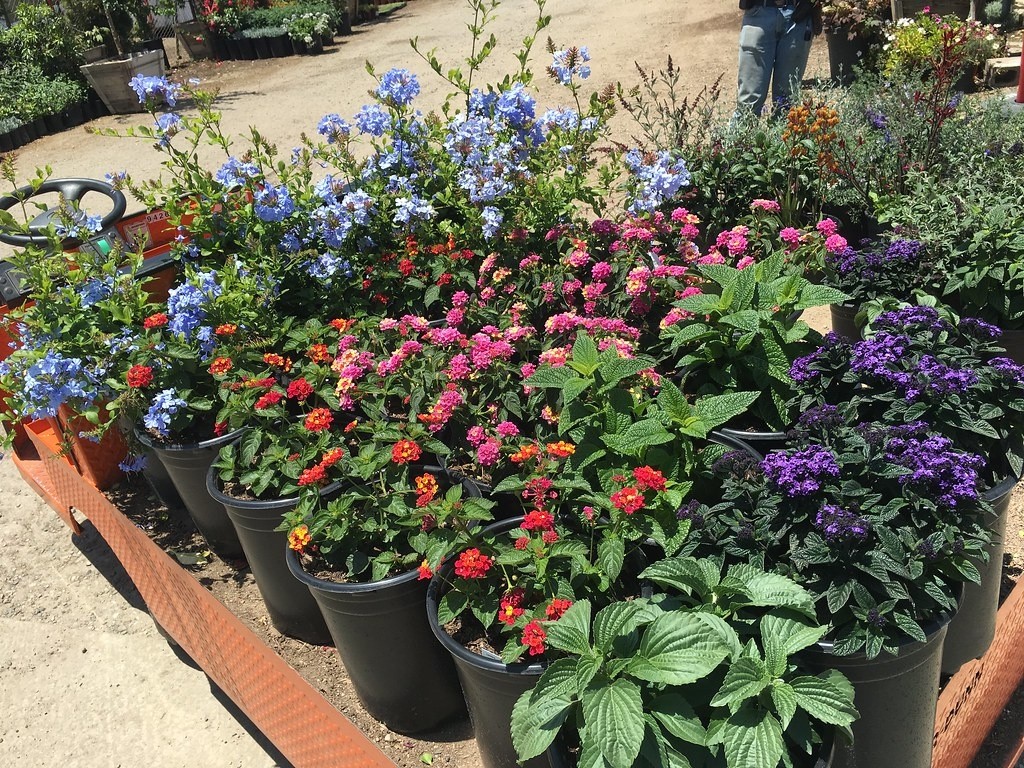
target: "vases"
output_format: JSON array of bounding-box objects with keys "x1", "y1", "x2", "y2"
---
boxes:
[{"x1": 110, "y1": 372, "x2": 1018, "y2": 767}]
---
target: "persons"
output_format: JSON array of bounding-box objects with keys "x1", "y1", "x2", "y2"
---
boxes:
[{"x1": 728, "y1": 0, "x2": 822, "y2": 137}]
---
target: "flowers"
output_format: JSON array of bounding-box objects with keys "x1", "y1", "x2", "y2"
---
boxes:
[{"x1": 0, "y1": 1, "x2": 1023, "y2": 655}]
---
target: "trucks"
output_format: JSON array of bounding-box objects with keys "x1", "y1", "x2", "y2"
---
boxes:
[{"x1": 0, "y1": 179, "x2": 1024, "y2": 768}]
